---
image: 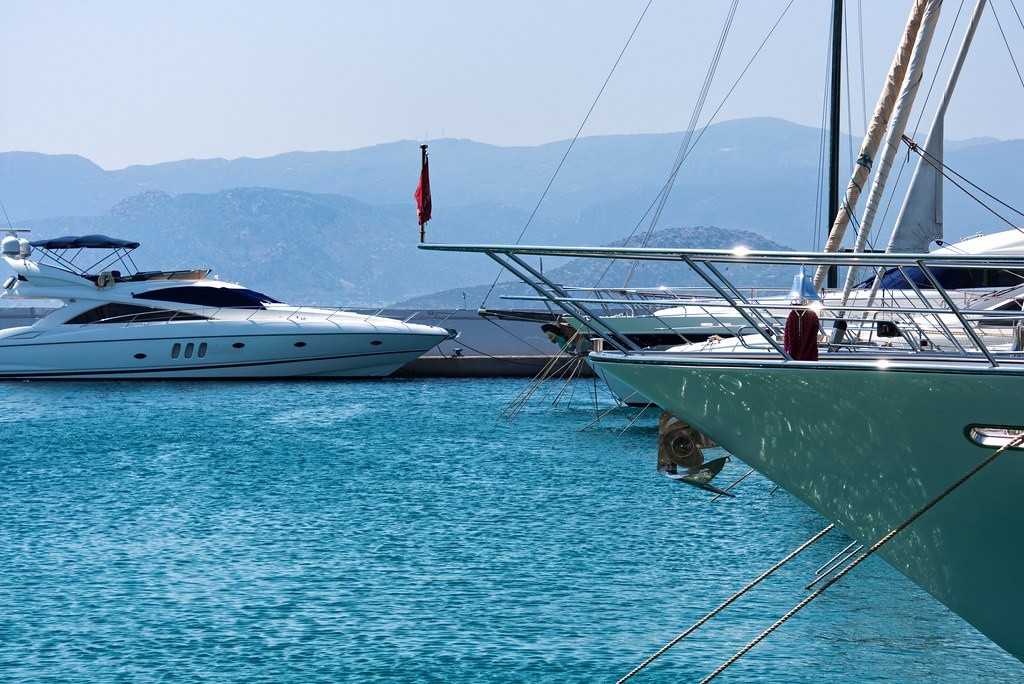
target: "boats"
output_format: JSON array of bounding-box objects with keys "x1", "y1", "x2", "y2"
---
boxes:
[
  {"x1": 0, "y1": 204, "x2": 459, "y2": 376},
  {"x1": 414, "y1": 0, "x2": 1024, "y2": 661}
]
